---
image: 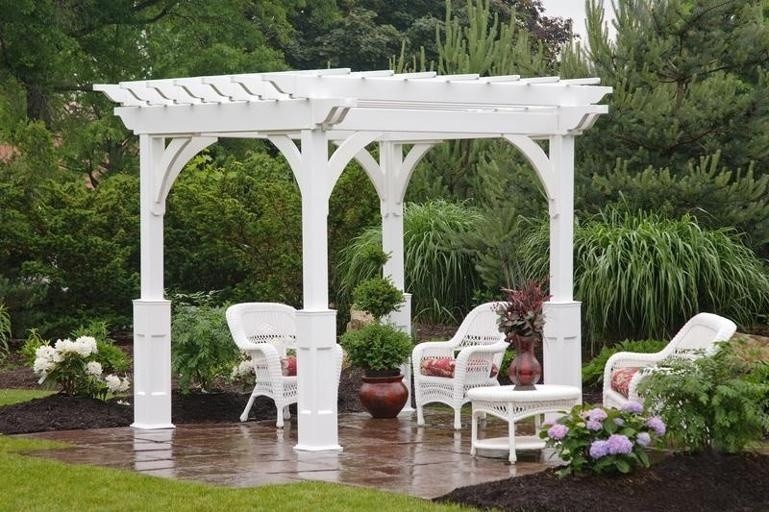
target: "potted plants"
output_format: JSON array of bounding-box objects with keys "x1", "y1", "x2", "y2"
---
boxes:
[{"x1": 340, "y1": 323, "x2": 413, "y2": 419}]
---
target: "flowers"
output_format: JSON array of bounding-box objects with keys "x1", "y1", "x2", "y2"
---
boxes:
[{"x1": 494, "y1": 273, "x2": 555, "y2": 341}]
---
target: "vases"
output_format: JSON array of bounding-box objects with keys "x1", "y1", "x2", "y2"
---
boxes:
[{"x1": 509, "y1": 335, "x2": 541, "y2": 391}]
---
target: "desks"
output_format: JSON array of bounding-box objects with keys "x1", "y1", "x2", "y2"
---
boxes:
[{"x1": 468, "y1": 384, "x2": 580, "y2": 464}]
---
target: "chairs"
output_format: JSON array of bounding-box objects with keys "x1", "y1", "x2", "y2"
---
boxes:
[
  {"x1": 411, "y1": 301, "x2": 519, "y2": 431},
  {"x1": 602, "y1": 311, "x2": 740, "y2": 409},
  {"x1": 225, "y1": 300, "x2": 344, "y2": 428}
]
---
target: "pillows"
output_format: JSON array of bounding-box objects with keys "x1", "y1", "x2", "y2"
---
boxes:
[
  {"x1": 250, "y1": 356, "x2": 297, "y2": 377},
  {"x1": 611, "y1": 365, "x2": 644, "y2": 398},
  {"x1": 417, "y1": 355, "x2": 499, "y2": 378}
]
